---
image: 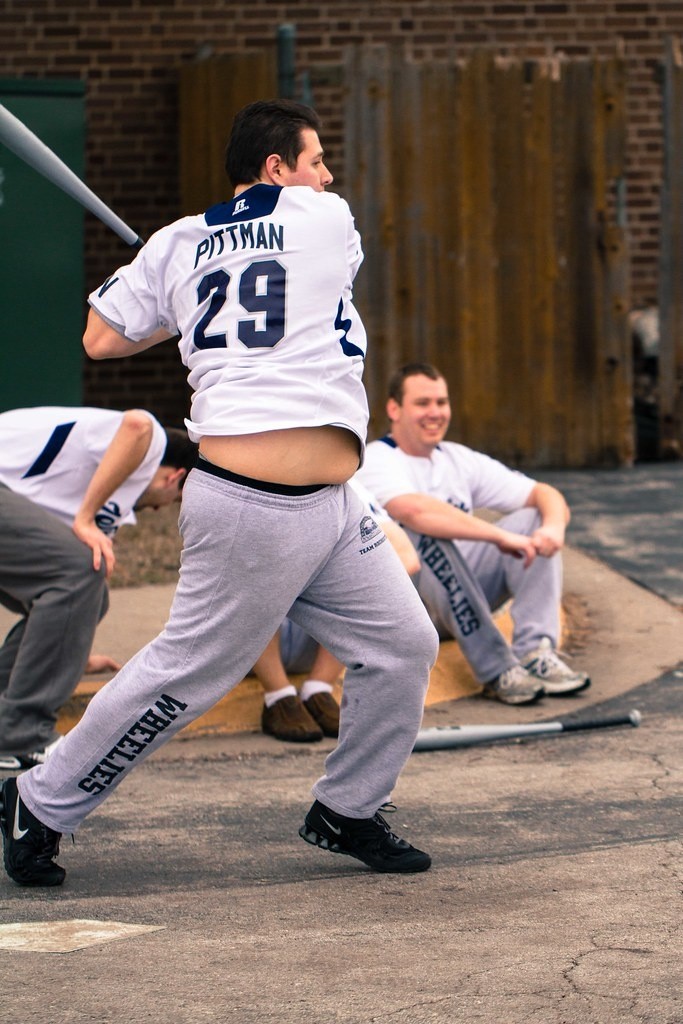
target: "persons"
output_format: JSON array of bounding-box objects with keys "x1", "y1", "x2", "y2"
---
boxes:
[
  {"x1": 0, "y1": 97, "x2": 439, "y2": 887},
  {"x1": 345, "y1": 363, "x2": 591, "y2": 704},
  {"x1": 627, "y1": 297, "x2": 661, "y2": 405}
]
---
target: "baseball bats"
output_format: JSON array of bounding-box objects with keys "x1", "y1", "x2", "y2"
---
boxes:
[
  {"x1": 0, "y1": 103, "x2": 145, "y2": 253},
  {"x1": 411, "y1": 708, "x2": 643, "y2": 751}
]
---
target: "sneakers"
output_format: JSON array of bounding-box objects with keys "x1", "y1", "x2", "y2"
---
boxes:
[
  {"x1": 0, "y1": 777, "x2": 66, "y2": 886},
  {"x1": 482, "y1": 666, "x2": 545, "y2": 706},
  {"x1": 516, "y1": 639, "x2": 590, "y2": 696},
  {"x1": 298, "y1": 802, "x2": 432, "y2": 872}
]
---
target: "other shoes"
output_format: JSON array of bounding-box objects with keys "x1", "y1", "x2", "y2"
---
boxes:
[
  {"x1": 301, "y1": 693, "x2": 340, "y2": 740},
  {"x1": 261, "y1": 695, "x2": 323, "y2": 742},
  {"x1": 0, "y1": 736, "x2": 63, "y2": 769}
]
